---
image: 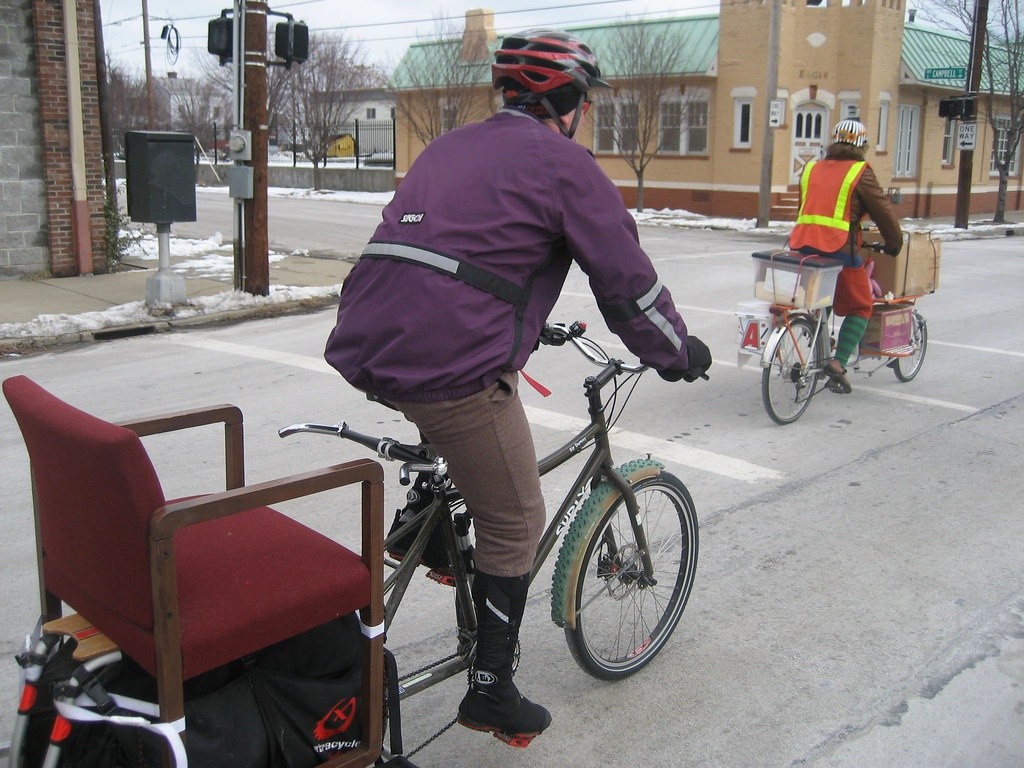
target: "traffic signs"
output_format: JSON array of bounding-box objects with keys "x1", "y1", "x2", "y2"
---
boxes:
[{"x1": 957, "y1": 124, "x2": 976, "y2": 149}]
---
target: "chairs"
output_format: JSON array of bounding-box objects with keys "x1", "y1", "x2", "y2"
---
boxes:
[{"x1": 3, "y1": 376, "x2": 386, "y2": 768}]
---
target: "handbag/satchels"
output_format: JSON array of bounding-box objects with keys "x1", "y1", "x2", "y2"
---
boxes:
[{"x1": 22, "y1": 611, "x2": 400, "y2": 768}]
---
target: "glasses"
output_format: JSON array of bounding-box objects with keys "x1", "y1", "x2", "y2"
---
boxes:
[{"x1": 580, "y1": 98, "x2": 592, "y2": 117}]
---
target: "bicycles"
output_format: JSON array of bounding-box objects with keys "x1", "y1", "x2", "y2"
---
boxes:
[{"x1": 760, "y1": 241, "x2": 928, "y2": 424}]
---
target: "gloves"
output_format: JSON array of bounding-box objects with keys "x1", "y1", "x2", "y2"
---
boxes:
[
  {"x1": 884, "y1": 244, "x2": 901, "y2": 256},
  {"x1": 658, "y1": 335, "x2": 712, "y2": 383}
]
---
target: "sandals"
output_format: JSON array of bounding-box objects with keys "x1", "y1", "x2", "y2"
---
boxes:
[
  {"x1": 808, "y1": 336, "x2": 835, "y2": 348},
  {"x1": 822, "y1": 359, "x2": 852, "y2": 394}
]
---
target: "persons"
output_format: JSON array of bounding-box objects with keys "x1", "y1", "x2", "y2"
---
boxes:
[
  {"x1": 788, "y1": 120, "x2": 904, "y2": 394},
  {"x1": 323, "y1": 28, "x2": 712, "y2": 739}
]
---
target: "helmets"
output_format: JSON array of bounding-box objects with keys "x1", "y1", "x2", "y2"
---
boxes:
[
  {"x1": 832, "y1": 120, "x2": 867, "y2": 147},
  {"x1": 492, "y1": 28, "x2": 612, "y2": 105}
]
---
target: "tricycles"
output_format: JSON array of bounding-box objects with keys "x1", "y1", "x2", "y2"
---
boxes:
[{"x1": 3, "y1": 321, "x2": 709, "y2": 768}]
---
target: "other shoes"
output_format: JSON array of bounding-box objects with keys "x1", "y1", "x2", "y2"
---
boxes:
[
  {"x1": 457, "y1": 674, "x2": 553, "y2": 739},
  {"x1": 387, "y1": 509, "x2": 476, "y2": 580}
]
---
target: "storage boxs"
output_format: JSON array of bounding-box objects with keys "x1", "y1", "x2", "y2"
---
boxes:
[
  {"x1": 861, "y1": 230, "x2": 940, "y2": 298},
  {"x1": 750, "y1": 248, "x2": 844, "y2": 312},
  {"x1": 859, "y1": 304, "x2": 915, "y2": 352}
]
